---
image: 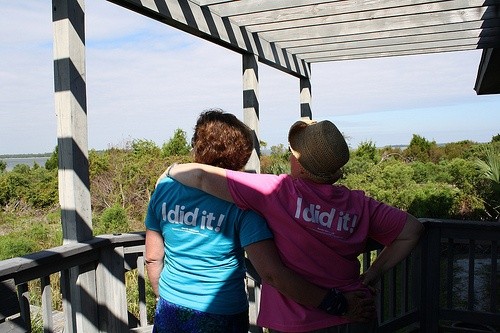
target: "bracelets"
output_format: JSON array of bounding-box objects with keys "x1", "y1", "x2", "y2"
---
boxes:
[{"x1": 316, "y1": 288, "x2": 350, "y2": 316}]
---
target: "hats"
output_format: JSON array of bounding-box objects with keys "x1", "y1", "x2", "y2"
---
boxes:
[{"x1": 288, "y1": 117, "x2": 350, "y2": 185}]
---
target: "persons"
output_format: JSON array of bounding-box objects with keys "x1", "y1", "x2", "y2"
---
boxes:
[
  {"x1": 167, "y1": 120, "x2": 421, "y2": 333},
  {"x1": 144, "y1": 110, "x2": 373, "y2": 333}
]
